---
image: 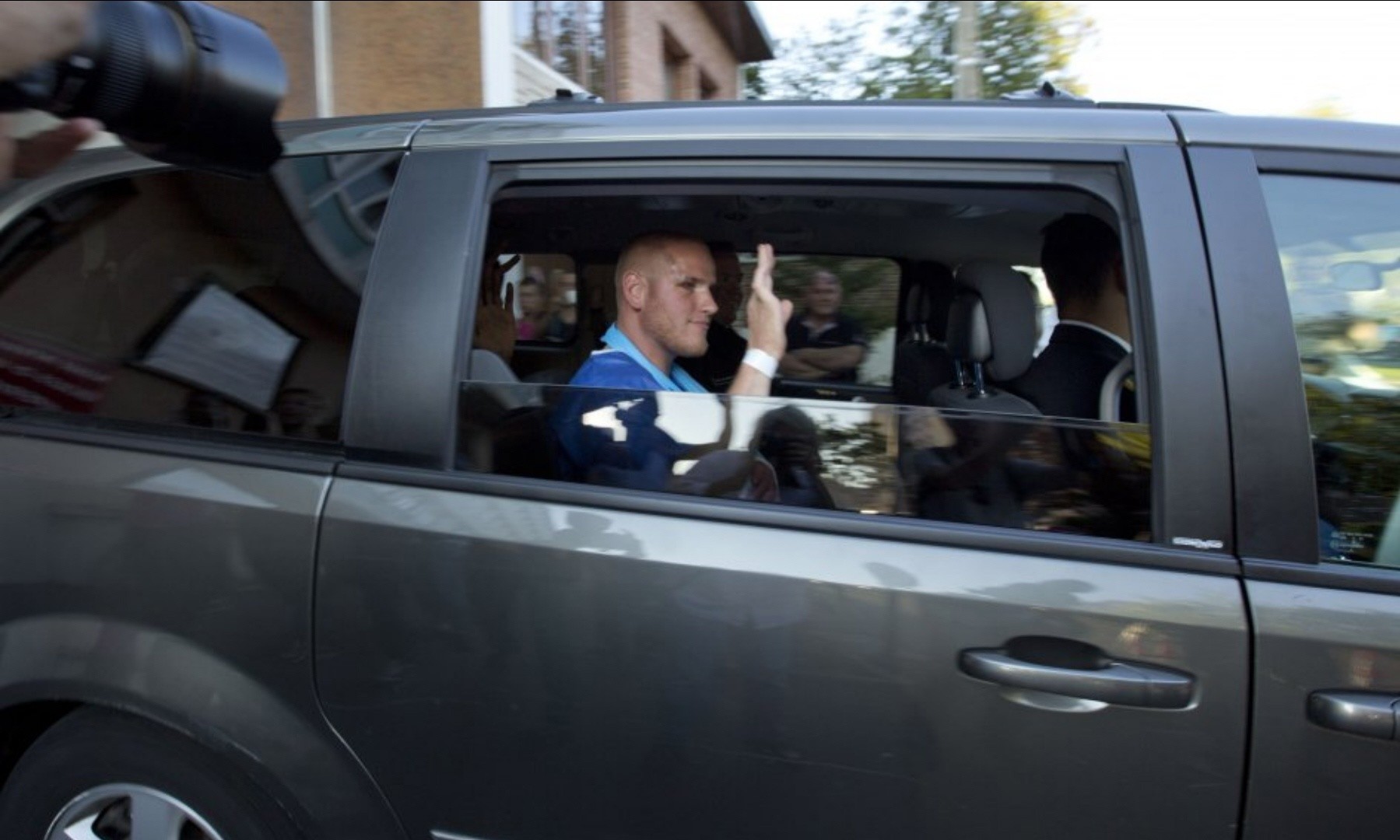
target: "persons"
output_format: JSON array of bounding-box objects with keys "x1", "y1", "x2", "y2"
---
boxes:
[
  {"x1": 0, "y1": 1, "x2": 100, "y2": 190},
  {"x1": 1030, "y1": 213, "x2": 1153, "y2": 542},
  {"x1": 461, "y1": 233, "x2": 866, "y2": 509}
]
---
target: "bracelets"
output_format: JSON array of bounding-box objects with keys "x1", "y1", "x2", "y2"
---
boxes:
[{"x1": 741, "y1": 347, "x2": 779, "y2": 379}]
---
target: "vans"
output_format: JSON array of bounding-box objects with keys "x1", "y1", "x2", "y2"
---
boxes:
[{"x1": 0, "y1": 102, "x2": 1400, "y2": 840}]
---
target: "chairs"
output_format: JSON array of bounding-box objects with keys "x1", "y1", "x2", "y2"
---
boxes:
[
  {"x1": 460, "y1": 350, "x2": 555, "y2": 471},
  {"x1": 900, "y1": 264, "x2": 1058, "y2": 427}
]
